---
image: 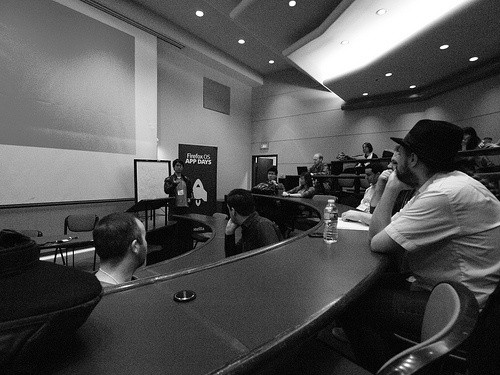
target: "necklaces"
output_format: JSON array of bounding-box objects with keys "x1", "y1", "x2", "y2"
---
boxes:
[{"x1": 99, "y1": 268, "x2": 119, "y2": 284}]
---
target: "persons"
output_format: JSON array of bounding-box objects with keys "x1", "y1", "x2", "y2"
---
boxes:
[
  {"x1": 336, "y1": 152, "x2": 356, "y2": 160},
  {"x1": 309, "y1": 153, "x2": 332, "y2": 194},
  {"x1": 460, "y1": 127, "x2": 481, "y2": 149},
  {"x1": 344, "y1": 143, "x2": 378, "y2": 174},
  {"x1": 333, "y1": 118, "x2": 500, "y2": 375},
  {"x1": 223, "y1": 188, "x2": 278, "y2": 258},
  {"x1": 92, "y1": 213, "x2": 148, "y2": 286},
  {"x1": 255, "y1": 168, "x2": 285, "y2": 208},
  {"x1": 274, "y1": 171, "x2": 315, "y2": 238},
  {"x1": 164, "y1": 159, "x2": 193, "y2": 221},
  {"x1": 340, "y1": 146, "x2": 415, "y2": 226}
]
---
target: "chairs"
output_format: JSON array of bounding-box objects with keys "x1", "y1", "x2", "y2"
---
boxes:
[
  {"x1": 63, "y1": 214, "x2": 99, "y2": 271},
  {"x1": 270, "y1": 156, "x2": 500, "y2": 375},
  {"x1": 19, "y1": 230, "x2": 42, "y2": 253}
]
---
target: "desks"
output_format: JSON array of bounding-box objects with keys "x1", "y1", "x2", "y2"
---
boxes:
[
  {"x1": 31, "y1": 236, "x2": 93, "y2": 249},
  {"x1": 133, "y1": 212, "x2": 243, "y2": 281},
  {"x1": 286, "y1": 171, "x2": 500, "y2": 190},
  {"x1": 0, "y1": 190, "x2": 392, "y2": 375},
  {"x1": 331, "y1": 147, "x2": 500, "y2": 191}
]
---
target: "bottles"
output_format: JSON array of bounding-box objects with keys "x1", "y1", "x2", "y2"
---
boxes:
[{"x1": 323, "y1": 199, "x2": 338, "y2": 245}]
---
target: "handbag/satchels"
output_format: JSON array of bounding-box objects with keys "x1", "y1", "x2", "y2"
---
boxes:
[{"x1": 0, "y1": 228, "x2": 104, "y2": 366}]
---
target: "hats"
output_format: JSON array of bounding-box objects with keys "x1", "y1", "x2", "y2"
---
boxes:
[{"x1": 389, "y1": 119, "x2": 464, "y2": 165}]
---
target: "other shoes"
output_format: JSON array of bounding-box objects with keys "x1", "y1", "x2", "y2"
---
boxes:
[{"x1": 332, "y1": 328, "x2": 351, "y2": 344}]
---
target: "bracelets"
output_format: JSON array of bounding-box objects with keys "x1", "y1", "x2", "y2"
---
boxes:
[{"x1": 289, "y1": 193, "x2": 290, "y2": 196}]
